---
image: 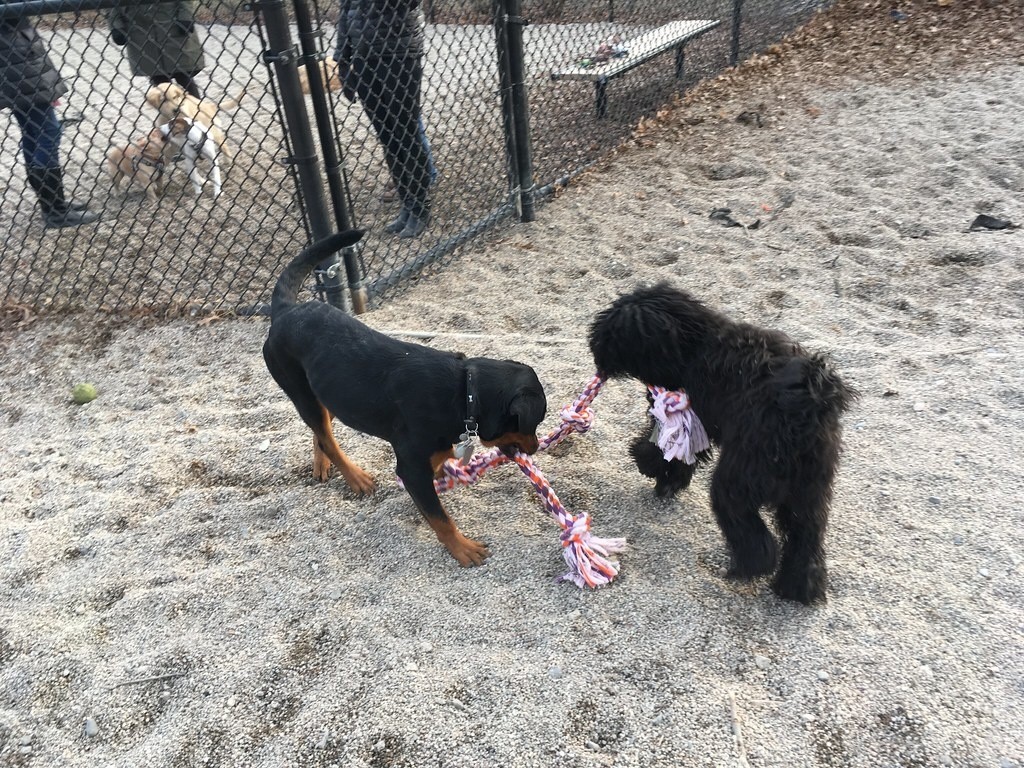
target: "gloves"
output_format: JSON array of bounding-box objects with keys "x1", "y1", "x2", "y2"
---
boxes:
[
  {"x1": 179, "y1": 21, "x2": 194, "y2": 38},
  {"x1": 339, "y1": 63, "x2": 357, "y2": 104},
  {"x1": 112, "y1": 29, "x2": 126, "y2": 45}
]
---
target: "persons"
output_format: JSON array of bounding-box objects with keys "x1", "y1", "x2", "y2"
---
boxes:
[
  {"x1": 334, "y1": 0, "x2": 438, "y2": 238},
  {"x1": 108, "y1": 0, "x2": 205, "y2": 97},
  {"x1": 0, "y1": 0, "x2": 96, "y2": 228}
]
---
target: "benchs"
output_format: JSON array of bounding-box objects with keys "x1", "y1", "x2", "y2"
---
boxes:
[{"x1": 549, "y1": 19, "x2": 724, "y2": 120}]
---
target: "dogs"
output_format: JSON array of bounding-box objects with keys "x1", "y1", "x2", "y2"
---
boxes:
[
  {"x1": 589, "y1": 281, "x2": 864, "y2": 605},
  {"x1": 297, "y1": 56, "x2": 354, "y2": 96},
  {"x1": 106, "y1": 82, "x2": 256, "y2": 208},
  {"x1": 262, "y1": 227, "x2": 548, "y2": 569}
]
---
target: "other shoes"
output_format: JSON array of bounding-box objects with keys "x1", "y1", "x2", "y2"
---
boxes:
[
  {"x1": 396, "y1": 210, "x2": 430, "y2": 238},
  {"x1": 60, "y1": 201, "x2": 87, "y2": 212},
  {"x1": 46, "y1": 205, "x2": 96, "y2": 226},
  {"x1": 386, "y1": 205, "x2": 412, "y2": 232}
]
